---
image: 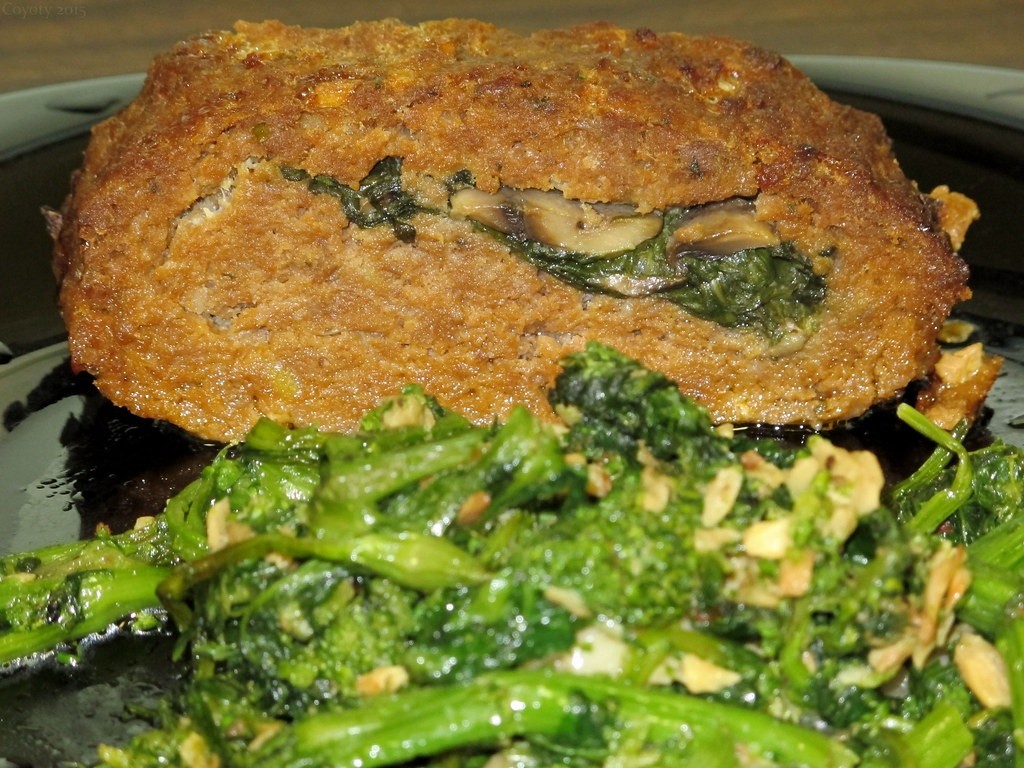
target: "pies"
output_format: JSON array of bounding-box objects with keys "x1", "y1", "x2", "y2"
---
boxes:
[{"x1": 60, "y1": 20, "x2": 957, "y2": 444}]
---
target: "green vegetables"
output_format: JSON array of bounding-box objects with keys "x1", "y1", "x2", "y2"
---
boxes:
[{"x1": 1, "y1": 343, "x2": 1024, "y2": 768}]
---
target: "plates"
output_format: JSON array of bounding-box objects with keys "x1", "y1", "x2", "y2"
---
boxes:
[{"x1": 0, "y1": 54, "x2": 1024, "y2": 768}]
